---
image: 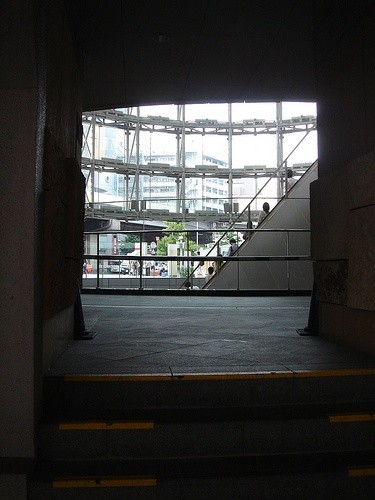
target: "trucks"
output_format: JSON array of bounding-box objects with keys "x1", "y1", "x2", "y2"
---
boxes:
[{"x1": 111, "y1": 260, "x2": 133, "y2": 275}]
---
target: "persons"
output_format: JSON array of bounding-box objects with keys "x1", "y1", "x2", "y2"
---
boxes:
[
  {"x1": 243, "y1": 222, "x2": 252, "y2": 241},
  {"x1": 205, "y1": 267, "x2": 214, "y2": 283},
  {"x1": 185, "y1": 282, "x2": 199, "y2": 290},
  {"x1": 287, "y1": 169, "x2": 297, "y2": 191},
  {"x1": 258, "y1": 202, "x2": 270, "y2": 225},
  {"x1": 227, "y1": 238, "x2": 238, "y2": 256},
  {"x1": 131, "y1": 261, "x2": 167, "y2": 276}
]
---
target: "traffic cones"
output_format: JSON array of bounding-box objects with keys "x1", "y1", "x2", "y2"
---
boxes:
[
  {"x1": 74, "y1": 278, "x2": 96, "y2": 341},
  {"x1": 297, "y1": 276, "x2": 319, "y2": 336}
]
